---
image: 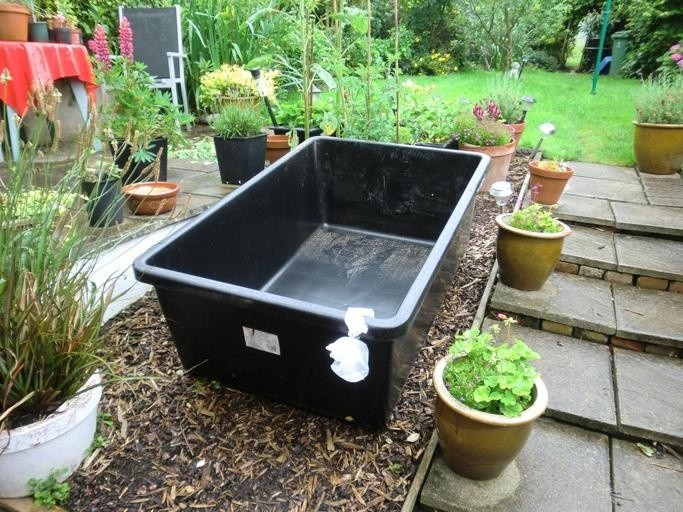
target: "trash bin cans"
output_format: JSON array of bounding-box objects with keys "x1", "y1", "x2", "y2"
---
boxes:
[{"x1": 609, "y1": 30, "x2": 634, "y2": 78}]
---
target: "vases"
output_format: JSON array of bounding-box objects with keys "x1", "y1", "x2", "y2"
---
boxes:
[
  {"x1": 108, "y1": 137, "x2": 167, "y2": 185},
  {"x1": 433, "y1": 354, "x2": 546, "y2": 480},
  {"x1": 458, "y1": 139, "x2": 515, "y2": 192},
  {"x1": 495, "y1": 213, "x2": 571, "y2": 291},
  {"x1": 267, "y1": 135, "x2": 299, "y2": 164},
  {"x1": 220, "y1": 95, "x2": 259, "y2": 112}
]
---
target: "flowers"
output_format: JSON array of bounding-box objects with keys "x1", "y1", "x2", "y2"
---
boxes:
[
  {"x1": 87, "y1": 16, "x2": 196, "y2": 140},
  {"x1": 444, "y1": 313, "x2": 540, "y2": 418},
  {"x1": 200, "y1": 63, "x2": 279, "y2": 109},
  {"x1": 451, "y1": 98, "x2": 512, "y2": 147},
  {"x1": 510, "y1": 182, "x2": 562, "y2": 233}
]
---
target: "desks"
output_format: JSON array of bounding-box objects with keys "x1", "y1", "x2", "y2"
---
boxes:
[{"x1": 0, "y1": 41, "x2": 100, "y2": 162}]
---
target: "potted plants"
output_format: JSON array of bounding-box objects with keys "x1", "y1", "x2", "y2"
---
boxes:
[
  {"x1": 210, "y1": 103, "x2": 267, "y2": 185},
  {"x1": 0, "y1": 0, "x2": 80, "y2": 46},
  {"x1": 0, "y1": 68, "x2": 124, "y2": 497},
  {"x1": 269, "y1": 100, "x2": 339, "y2": 144},
  {"x1": 484, "y1": 93, "x2": 537, "y2": 154},
  {"x1": 632, "y1": 72, "x2": 683, "y2": 174},
  {"x1": 529, "y1": 162, "x2": 573, "y2": 204}
]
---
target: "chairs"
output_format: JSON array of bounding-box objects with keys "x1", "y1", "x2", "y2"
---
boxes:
[{"x1": 109, "y1": 7, "x2": 192, "y2": 134}]
